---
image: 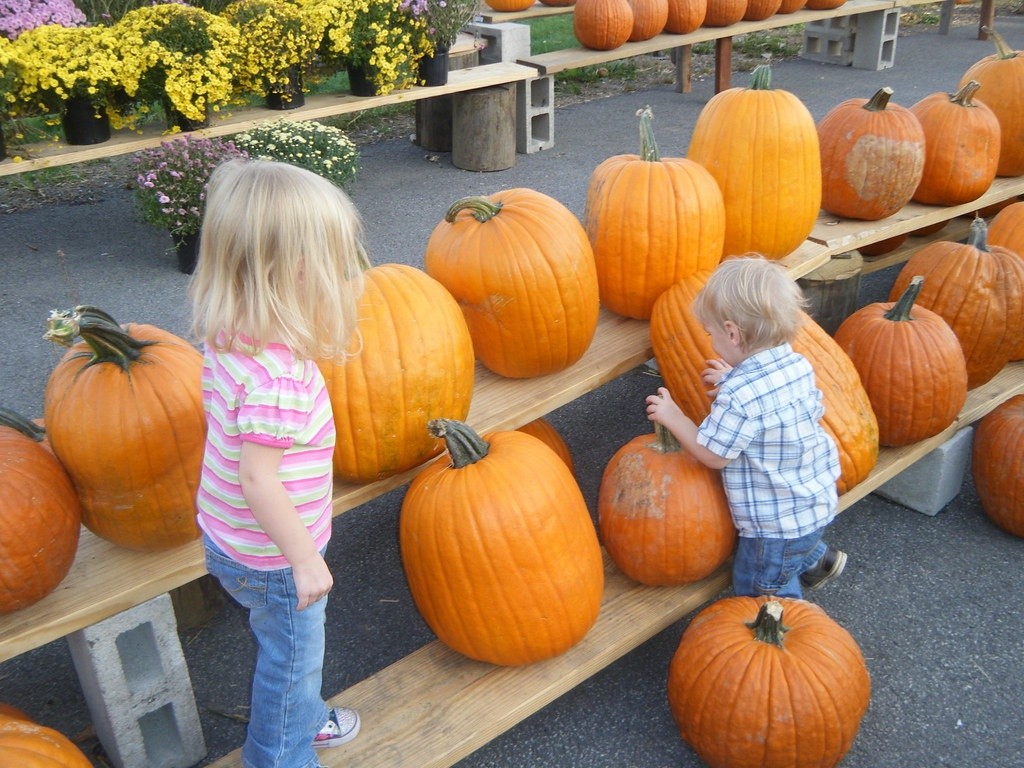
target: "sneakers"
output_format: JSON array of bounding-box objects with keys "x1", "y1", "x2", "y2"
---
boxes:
[
  {"x1": 312, "y1": 705, "x2": 361, "y2": 750},
  {"x1": 798, "y1": 545, "x2": 847, "y2": 592}
]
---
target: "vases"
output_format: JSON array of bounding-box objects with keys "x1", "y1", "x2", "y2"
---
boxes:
[
  {"x1": 58, "y1": 99, "x2": 111, "y2": 145},
  {"x1": 168, "y1": 226, "x2": 202, "y2": 275},
  {"x1": 346, "y1": 58, "x2": 380, "y2": 97},
  {"x1": 412, "y1": 40, "x2": 450, "y2": 87},
  {"x1": 262, "y1": 63, "x2": 304, "y2": 110},
  {"x1": 160, "y1": 82, "x2": 210, "y2": 132}
]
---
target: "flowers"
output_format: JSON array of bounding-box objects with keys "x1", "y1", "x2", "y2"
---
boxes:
[
  {"x1": 295, "y1": 0, "x2": 436, "y2": 98},
  {"x1": 234, "y1": 116, "x2": 364, "y2": 193},
  {"x1": 115, "y1": 2, "x2": 250, "y2": 123},
  {"x1": 12, "y1": 23, "x2": 145, "y2": 136},
  {"x1": 217, "y1": 0, "x2": 323, "y2": 103},
  {"x1": 129, "y1": 133, "x2": 250, "y2": 256},
  {"x1": 399, "y1": 0, "x2": 486, "y2": 52}
]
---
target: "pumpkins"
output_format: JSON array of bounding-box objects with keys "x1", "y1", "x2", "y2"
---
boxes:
[
  {"x1": 42, "y1": 304, "x2": 215, "y2": 552},
  {"x1": 0, "y1": 405, "x2": 81, "y2": 614},
  {"x1": 299, "y1": 24, "x2": 1024, "y2": 664},
  {"x1": 0, "y1": 700, "x2": 96, "y2": 768},
  {"x1": 486, "y1": 0, "x2": 849, "y2": 50},
  {"x1": 666, "y1": 596, "x2": 870, "y2": 768}
]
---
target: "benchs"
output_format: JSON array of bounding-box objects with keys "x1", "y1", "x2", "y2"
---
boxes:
[{"x1": 0, "y1": 0, "x2": 1024, "y2": 768}]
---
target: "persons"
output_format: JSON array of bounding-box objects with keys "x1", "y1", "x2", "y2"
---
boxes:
[
  {"x1": 644, "y1": 258, "x2": 847, "y2": 600},
  {"x1": 194, "y1": 160, "x2": 363, "y2": 768}
]
